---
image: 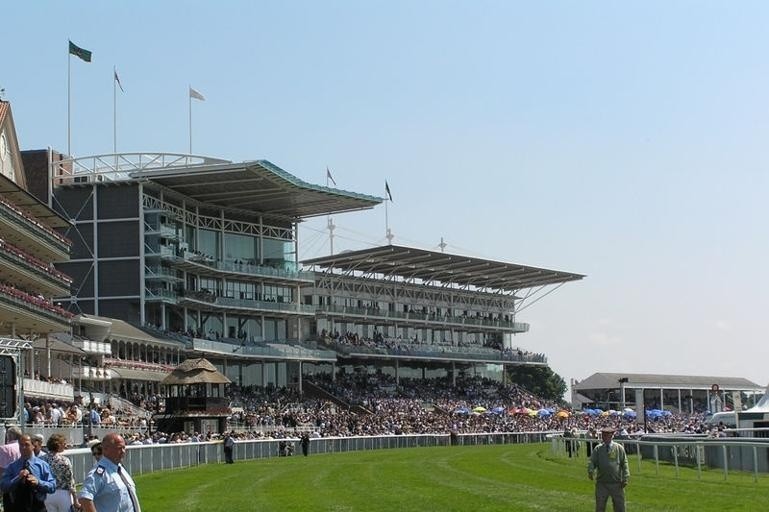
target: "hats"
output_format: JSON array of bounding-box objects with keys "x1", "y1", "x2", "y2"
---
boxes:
[{"x1": 602, "y1": 427, "x2": 615, "y2": 433}]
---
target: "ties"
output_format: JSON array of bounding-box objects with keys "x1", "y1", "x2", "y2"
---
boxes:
[
  {"x1": 605, "y1": 444, "x2": 609, "y2": 452},
  {"x1": 116, "y1": 466, "x2": 140, "y2": 512},
  {"x1": 23, "y1": 461, "x2": 29, "y2": 468}
]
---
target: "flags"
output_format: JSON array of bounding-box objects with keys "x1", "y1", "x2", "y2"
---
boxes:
[
  {"x1": 114, "y1": 72, "x2": 125, "y2": 92},
  {"x1": 69, "y1": 41, "x2": 92, "y2": 64},
  {"x1": 326, "y1": 169, "x2": 335, "y2": 184},
  {"x1": 385, "y1": 183, "x2": 395, "y2": 203},
  {"x1": 189, "y1": 88, "x2": 208, "y2": 103}
]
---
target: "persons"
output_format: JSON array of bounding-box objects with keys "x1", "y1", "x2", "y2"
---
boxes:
[
  {"x1": 0, "y1": 433, "x2": 56, "y2": 511},
  {"x1": 88, "y1": 439, "x2": 103, "y2": 461},
  {"x1": 592, "y1": 429, "x2": 599, "y2": 450},
  {"x1": 279, "y1": 440, "x2": 292, "y2": 456},
  {"x1": 40, "y1": 432, "x2": 81, "y2": 512},
  {"x1": 78, "y1": 432, "x2": 141, "y2": 512},
  {"x1": 588, "y1": 426, "x2": 630, "y2": 511},
  {"x1": 0, "y1": 195, "x2": 739, "y2": 449},
  {"x1": 299, "y1": 434, "x2": 310, "y2": 457},
  {"x1": 31, "y1": 434, "x2": 48, "y2": 453},
  {"x1": 0, "y1": 426, "x2": 23, "y2": 474},
  {"x1": 586, "y1": 426, "x2": 593, "y2": 457},
  {"x1": 33, "y1": 439, "x2": 47, "y2": 457},
  {"x1": 563, "y1": 426, "x2": 571, "y2": 459},
  {"x1": 223, "y1": 433, "x2": 233, "y2": 464},
  {"x1": 571, "y1": 428, "x2": 580, "y2": 456}
]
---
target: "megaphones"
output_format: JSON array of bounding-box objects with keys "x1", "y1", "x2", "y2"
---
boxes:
[{"x1": 621, "y1": 378, "x2": 629, "y2": 383}]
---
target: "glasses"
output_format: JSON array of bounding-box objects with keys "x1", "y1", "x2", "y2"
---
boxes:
[{"x1": 92, "y1": 451, "x2": 102, "y2": 456}]
